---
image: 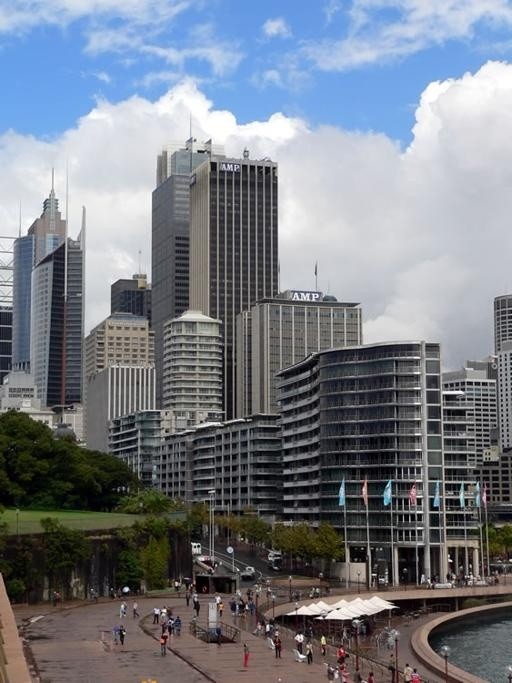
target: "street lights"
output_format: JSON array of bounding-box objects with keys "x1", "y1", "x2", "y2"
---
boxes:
[
  {"x1": 254, "y1": 580, "x2": 276, "y2": 624},
  {"x1": 288, "y1": 576, "x2": 293, "y2": 602},
  {"x1": 357, "y1": 571, "x2": 361, "y2": 593},
  {"x1": 353, "y1": 619, "x2": 360, "y2": 670},
  {"x1": 320, "y1": 609, "x2": 328, "y2": 638},
  {"x1": 392, "y1": 631, "x2": 401, "y2": 681},
  {"x1": 319, "y1": 573, "x2": 323, "y2": 598},
  {"x1": 294, "y1": 603, "x2": 299, "y2": 636},
  {"x1": 403, "y1": 568, "x2": 408, "y2": 590},
  {"x1": 440, "y1": 646, "x2": 451, "y2": 682}
]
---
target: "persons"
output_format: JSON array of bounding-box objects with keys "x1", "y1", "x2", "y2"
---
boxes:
[
  {"x1": 420, "y1": 569, "x2": 499, "y2": 590},
  {"x1": 113, "y1": 621, "x2": 120, "y2": 646},
  {"x1": 54, "y1": 591, "x2": 61, "y2": 608},
  {"x1": 90, "y1": 588, "x2": 99, "y2": 603},
  {"x1": 110, "y1": 588, "x2": 116, "y2": 599},
  {"x1": 123, "y1": 601, "x2": 128, "y2": 616},
  {"x1": 120, "y1": 601, "x2": 125, "y2": 619},
  {"x1": 133, "y1": 599, "x2": 140, "y2": 620},
  {"x1": 118, "y1": 588, "x2": 122, "y2": 597},
  {"x1": 118, "y1": 625, "x2": 127, "y2": 646},
  {"x1": 153, "y1": 575, "x2": 423, "y2": 682}
]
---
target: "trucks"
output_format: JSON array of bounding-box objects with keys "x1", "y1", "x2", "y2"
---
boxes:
[{"x1": 240, "y1": 566, "x2": 256, "y2": 581}]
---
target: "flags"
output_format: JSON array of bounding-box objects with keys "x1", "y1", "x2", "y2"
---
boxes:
[
  {"x1": 481, "y1": 481, "x2": 487, "y2": 511},
  {"x1": 338, "y1": 474, "x2": 346, "y2": 507},
  {"x1": 433, "y1": 479, "x2": 441, "y2": 508},
  {"x1": 362, "y1": 475, "x2": 368, "y2": 507},
  {"x1": 410, "y1": 478, "x2": 417, "y2": 507},
  {"x1": 475, "y1": 479, "x2": 481, "y2": 508},
  {"x1": 459, "y1": 479, "x2": 465, "y2": 508},
  {"x1": 383, "y1": 475, "x2": 392, "y2": 506}
]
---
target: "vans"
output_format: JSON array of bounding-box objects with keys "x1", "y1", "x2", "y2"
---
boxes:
[{"x1": 191, "y1": 543, "x2": 202, "y2": 555}]
